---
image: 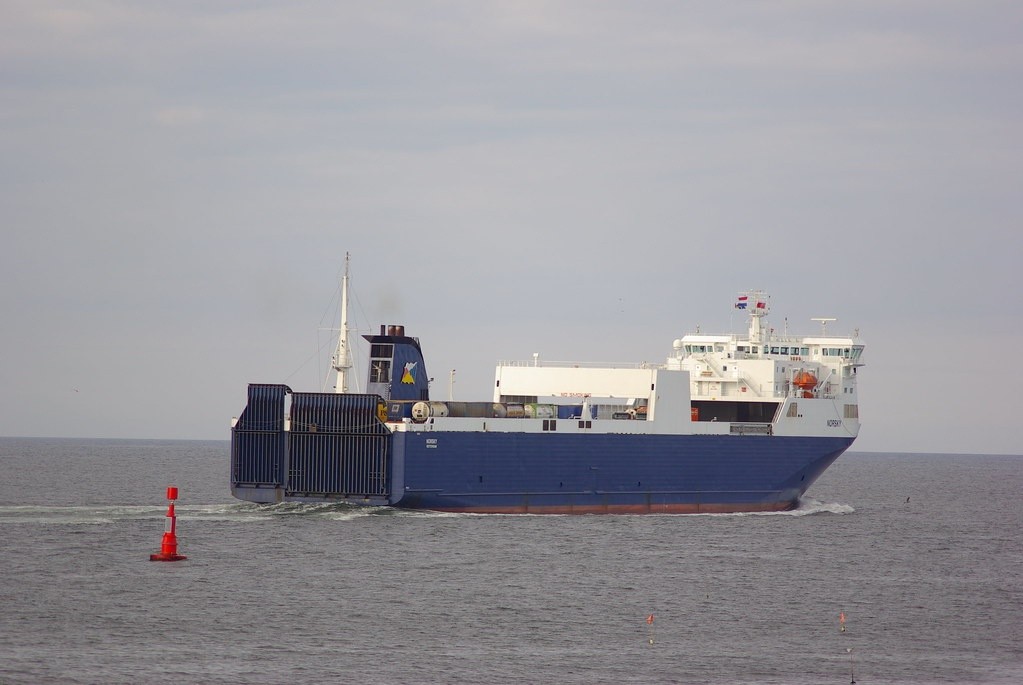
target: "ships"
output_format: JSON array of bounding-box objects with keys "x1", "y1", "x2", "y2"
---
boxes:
[{"x1": 227, "y1": 247, "x2": 867, "y2": 518}]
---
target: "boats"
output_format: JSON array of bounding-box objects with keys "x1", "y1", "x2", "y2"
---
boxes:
[{"x1": 792, "y1": 372, "x2": 817, "y2": 391}]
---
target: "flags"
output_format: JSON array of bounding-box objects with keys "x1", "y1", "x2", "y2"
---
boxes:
[
  {"x1": 738, "y1": 297, "x2": 747, "y2": 306},
  {"x1": 757, "y1": 303, "x2": 766, "y2": 308}
]
